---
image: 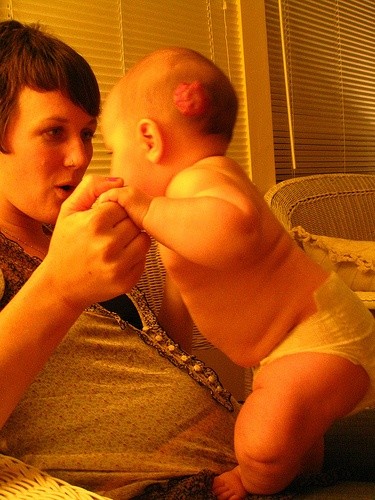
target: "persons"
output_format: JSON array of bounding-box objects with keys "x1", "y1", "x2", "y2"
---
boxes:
[
  {"x1": 92, "y1": 46, "x2": 375, "y2": 499},
  {"x1": 0, "y1": 20, "x2": 374, "y2": 498}
]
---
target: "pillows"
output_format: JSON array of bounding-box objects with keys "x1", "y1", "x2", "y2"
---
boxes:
[{"x1": 290, "y1": 222, "x2": 374, "y2": 293}]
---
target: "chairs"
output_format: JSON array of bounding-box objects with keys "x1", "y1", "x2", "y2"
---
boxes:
[{"x1": 261, "y1": 171, "x2": 374, "y2": 317}]
